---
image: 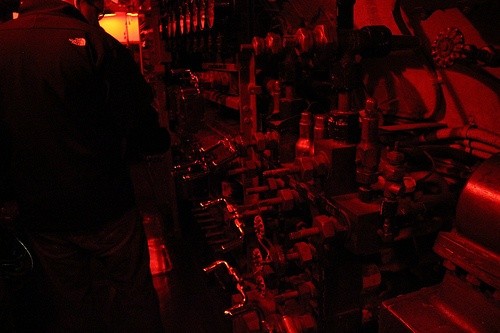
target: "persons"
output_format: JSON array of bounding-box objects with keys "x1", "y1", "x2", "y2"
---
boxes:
[{"x1": 0, "y1": 0, "x2": 172, "y2": 333}]
